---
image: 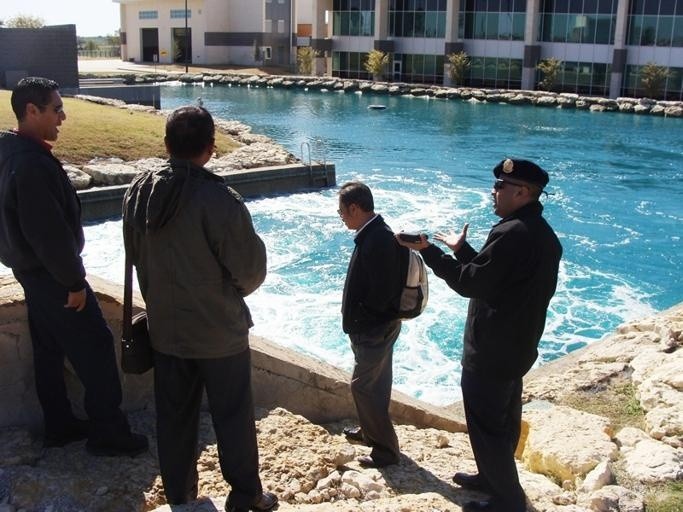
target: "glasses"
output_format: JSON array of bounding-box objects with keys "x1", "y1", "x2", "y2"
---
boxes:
[
  {"x1": 494, "y1": 178, "x2": 522, "y2": 189},
  {"x1": 35, "y1": 104, "x2": 63, "y2": 113}
]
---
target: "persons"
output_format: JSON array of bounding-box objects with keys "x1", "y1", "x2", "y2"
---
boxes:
[
  {"x1": 337, "y1": 178, "x2": 407, "y2": 469},
  {"x1": 391, "y1": 157, "x2": 562, "y2": 511},
  {"x1": 120, "y1": 105, "x2": 279, "y2": 511},
  {"x1": 0, "y1": 74, "x2": 149, "y2": 458}
]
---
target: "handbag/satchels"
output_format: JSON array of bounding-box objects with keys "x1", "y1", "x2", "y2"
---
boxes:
[
  {"x1": 398, "y1": 246, "x2": 429, "y2": 319},
  {"x1": 121, "y1": 311, "x2": 153, "y2": 373}
]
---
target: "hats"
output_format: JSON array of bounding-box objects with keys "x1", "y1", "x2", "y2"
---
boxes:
[{"x1": 493, "y1": 159, "x2": 549, "y2": 187}]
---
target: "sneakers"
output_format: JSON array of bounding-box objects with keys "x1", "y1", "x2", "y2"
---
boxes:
[
  {"x1": 45, "y1": 418, "x2": 91, "y2": 447},
  {"x1": 85, "y1": 433, "x2": 149, "y2": 456}
]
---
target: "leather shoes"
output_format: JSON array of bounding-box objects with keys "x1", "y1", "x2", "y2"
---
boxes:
[
  {"x1": 358, "y1": 456, "x2": 376, "y2": 467},
  {"x1": 462, "y1": 500, "x2": 527, "y2": 512},
  {"x1": 453, "y1": 473, "x2": 492, "y2": 492},
  {"x1": 225, "y1": 492, "x2": 279, "y2": 512},
  {"x1": 345, "y1": 426, "x2": 376, "y2": 446}
]
